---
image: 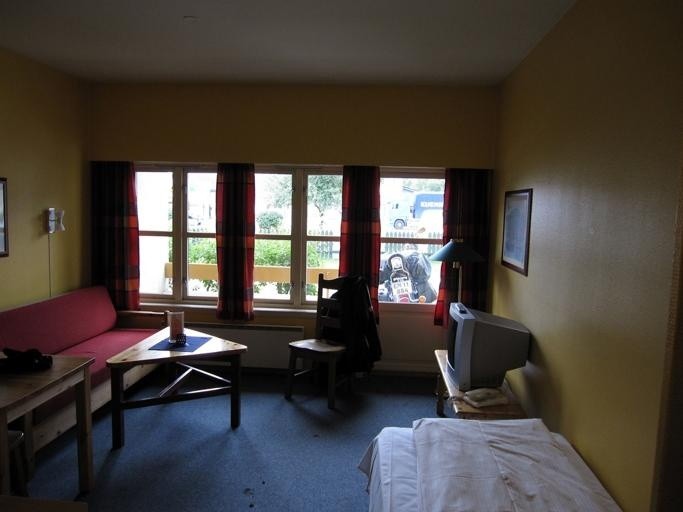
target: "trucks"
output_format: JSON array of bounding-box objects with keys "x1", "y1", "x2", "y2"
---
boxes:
[{"x1": 382, "y1": 193, "x2": 444, "y2": 234}]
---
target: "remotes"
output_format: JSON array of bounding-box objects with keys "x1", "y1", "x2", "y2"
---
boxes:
[{"x1": 455, "y1": 303, "x2": 467, "y2": 314}]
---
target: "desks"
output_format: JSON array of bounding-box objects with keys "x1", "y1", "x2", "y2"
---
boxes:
[
  {"x1": 107, "y1": 324, "x2": 249, "y2": 451},
  {"x1": 1, "y1": 350, "x2": 95, "y2": 512}
]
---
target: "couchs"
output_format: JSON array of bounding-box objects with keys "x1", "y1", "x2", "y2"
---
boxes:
[{"x1": 0, "y1": 286, "x2": 165, "y2": 456}]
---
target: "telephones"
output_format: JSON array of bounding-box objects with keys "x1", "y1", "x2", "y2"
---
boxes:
[{"x1": 462, "y1": 387, "x2": 509, "y2": 408}]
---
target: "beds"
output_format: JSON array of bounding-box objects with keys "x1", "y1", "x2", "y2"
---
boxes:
[{"x1": 371, "y1": 412, "x2": 627, "y2": 512}]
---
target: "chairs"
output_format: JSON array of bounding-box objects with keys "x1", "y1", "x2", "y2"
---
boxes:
[{"x1": 284, "y1": 272, "x2": 359, "y2": 409}]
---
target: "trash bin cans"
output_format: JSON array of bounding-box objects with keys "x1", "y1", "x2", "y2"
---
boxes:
[{"x1": 8, "y1": 430, "x2": 30, "y2": 498}]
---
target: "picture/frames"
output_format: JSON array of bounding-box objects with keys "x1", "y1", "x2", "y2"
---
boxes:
[{"x1": 500, "y1": 188, "x2": 533, "y2": 277}]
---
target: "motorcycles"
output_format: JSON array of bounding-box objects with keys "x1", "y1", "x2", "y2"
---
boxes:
[{"x1": 378, "y1": 255, "x2": 426, "y2": 304}]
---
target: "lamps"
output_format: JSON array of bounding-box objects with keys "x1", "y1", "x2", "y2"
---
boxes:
[
  {"x1": 437, "y1": 240, "x2": 485, "y2": 302},
  {"x1": 46, "y1": 208, "x2": 65, "y2": 235}
]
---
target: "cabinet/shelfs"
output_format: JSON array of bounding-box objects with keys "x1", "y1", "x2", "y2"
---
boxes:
[{"x1": 433, "y1": 346, "x2": 527, "y2": 418}]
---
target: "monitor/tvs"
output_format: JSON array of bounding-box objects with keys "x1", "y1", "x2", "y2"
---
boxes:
[{"x1": 446, "y1": 302, "x2": 530, "y2": 396}]
